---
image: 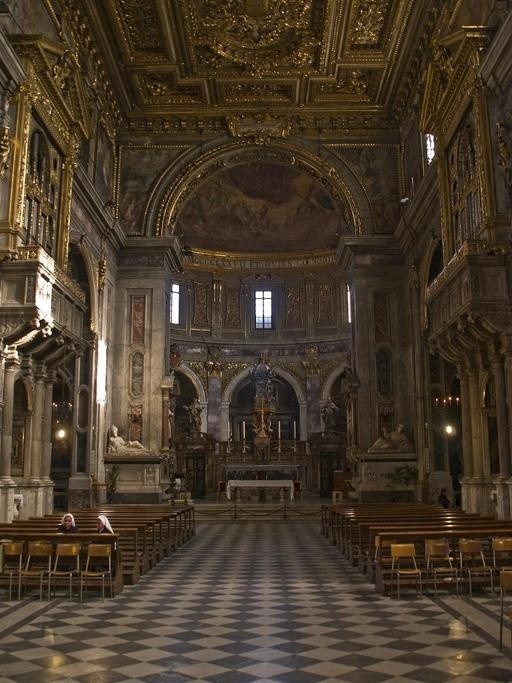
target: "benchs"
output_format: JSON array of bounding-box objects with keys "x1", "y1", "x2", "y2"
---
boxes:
[
  {"x1": 321, "y1": 502, "x2": 511, "y2": 596},
  {"x1": 0, "y1": 503, "x2": 195, "y2": 598}
]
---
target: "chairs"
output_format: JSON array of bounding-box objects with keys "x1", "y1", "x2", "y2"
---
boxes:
[{"x1": 390, "y1": 538, "x2": 512, "y2": 652}]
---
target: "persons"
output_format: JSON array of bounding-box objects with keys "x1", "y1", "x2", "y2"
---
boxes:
[
  {"x1": 231, "y1": 471, "x2": 239, "y2": 481},
  {"x1": 57, "y1": 513, "x2": 79, "y2": 533},
  {"x1": 107, "y1": 424, "x2": 152, "y2": 456},
  {"x1": 322, "y1": 395, "x2": 340, "y2": 437},
  {"x1": 95, "y1": 515, "x2": 117, "y2": 561},
  {"x1": 368, "y1": 423, "x2": 412, "y2": 450},
  {"x1": 183, "y1": 396, "x2": 204, "y2": 439},
  {"x1": 163, "y1": 397, "x2": 174, "y2": 447},
  {"x1": 238, "y1": 471, "x2": 244, "y2": 481},
  {"x1": 438, "y1": 487, "x2": 450, "y2": 510}
]
